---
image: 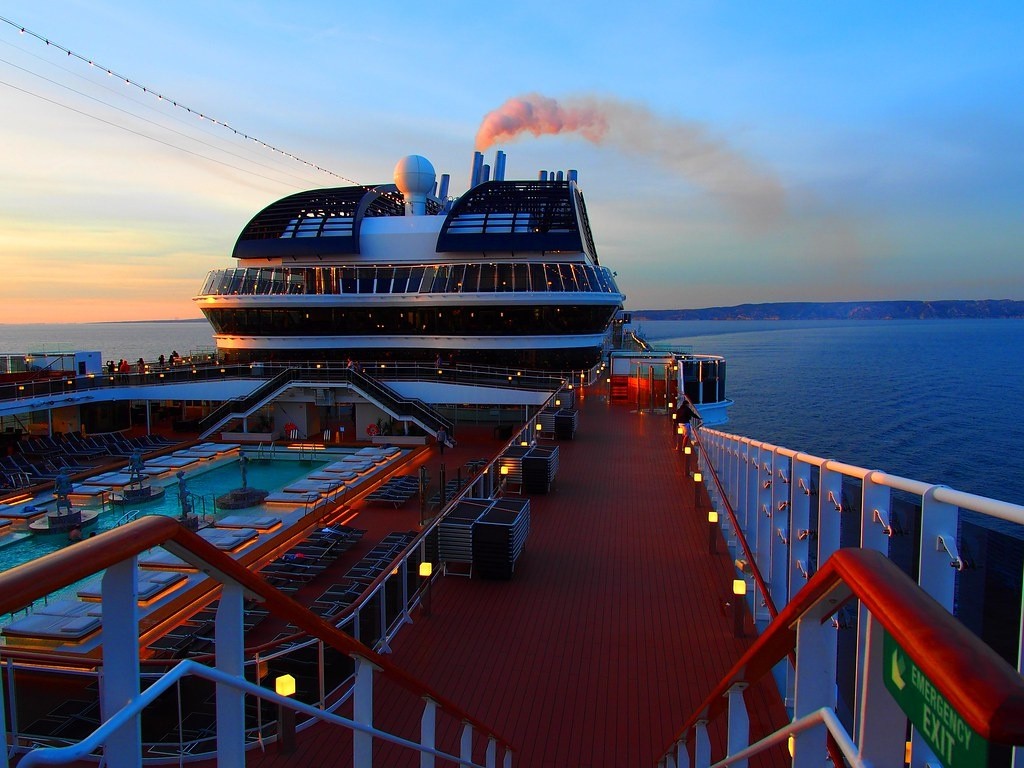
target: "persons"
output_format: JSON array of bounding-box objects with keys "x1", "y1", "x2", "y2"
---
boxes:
[
  {"x1": 436, "y1": 426, "x2": 448, "y2": 454},
  {"x1": 169, "y1": 351, "x2": 179, "y2": 366},
  {"x1": 158, "y1": 354, "x2": 165, "y2": 369},
  {"x1": 238, "y1": 451, "x2": 247, "y2": 491},
  {"x1": 435, "y1": 353, "x2": 443, "y2": 379},
  {"x1": 119, "y1": 360, "x2": 131, "y2": 384},
  {"x1": 53, "y1": 466, "x2": 74, "y2": 523},
  {"x1": 68, "y1": 523, "x2": 97, "y2": 546},
  {"x1": 128, "y1": 447, "x2": 145, "y2": 492},
  {"x1": 175, "y1": 470, "x2": 190, "y2": 520},
  {"x1": 117, "y1": 358, "x2": 123, "y2": 374},
  {"x1": 356, "y1": 360, "x2": 363, "y2": 377},
  {"x1": 23, "y1": 359, "x2": 28, "y2": 372},
  {"x1": 674, "y1": 400, "x2": 703, "y2": 452},
  {"x1": 346, "y1": 358, "x2": 354, "y2": 381},
  {"x1": 105, "y1": 360, "x2": 116, "y2": 375},
  {"x1": 448, "y1": 354, "x2": 456, "y2": 383},
  {"x1": 137, "y1": 357, "x2": 146, "y2": 384}
]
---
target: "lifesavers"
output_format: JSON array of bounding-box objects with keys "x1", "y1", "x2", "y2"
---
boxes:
[{"x1": 366, "y1": 424, "x2": 379, "y2": 436}]
